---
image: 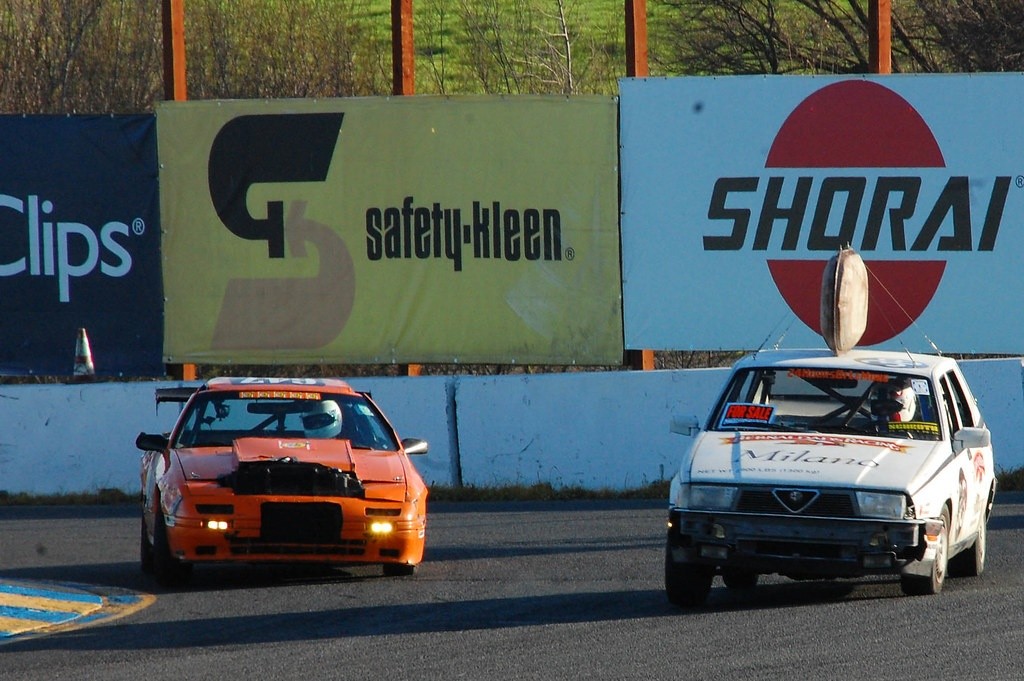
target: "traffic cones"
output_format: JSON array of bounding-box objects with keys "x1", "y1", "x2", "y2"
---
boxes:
[{"x1": 72, "y1": 327, "x2": 96, "y2": 378}]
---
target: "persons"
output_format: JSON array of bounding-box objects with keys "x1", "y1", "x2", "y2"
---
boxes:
[
  {"x1": 299, "y1": 400, "x2": 343, "y2": 439},
  {"x1": 858, "y1": 377, "x2": 923, "y2": 438}
]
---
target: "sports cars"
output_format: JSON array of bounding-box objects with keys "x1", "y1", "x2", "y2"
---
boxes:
[{"x1": 135, "y1": 377, "x2": 429, "y2": 595}]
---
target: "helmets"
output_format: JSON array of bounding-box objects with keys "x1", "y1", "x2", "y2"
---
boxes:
[
  {"x1": 299, "y1": 400, "x2": 342, "y2": 438},
  {"x1": 870, "y1": 378, "x2": 917, "y2": 422}
]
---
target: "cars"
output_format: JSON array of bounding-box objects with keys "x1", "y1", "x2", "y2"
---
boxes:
[{"x1": 662, "y1": 348, "x2": 998, "y2": 605}]
